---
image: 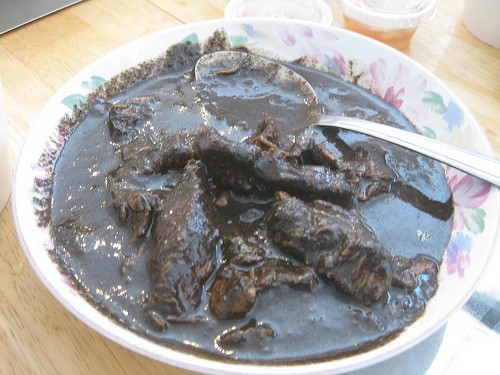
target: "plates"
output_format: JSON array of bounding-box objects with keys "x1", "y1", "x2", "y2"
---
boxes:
[
  {"x1": 10, "y1": 16, "x2": 497, "y2": 374},
  {"x1": 224, "y1": 0, "x2": 333, "y2": 26}
]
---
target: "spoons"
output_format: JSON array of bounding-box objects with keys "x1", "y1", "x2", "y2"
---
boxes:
[{"x1": 196, "y1": 49, "x2": 499, "y2": 195}]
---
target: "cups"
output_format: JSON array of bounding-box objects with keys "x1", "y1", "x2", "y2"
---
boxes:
[
  {"x1": 462, "y1": 0, "x2": 500, "y2": 49},
  {"x1": 343, "y1": 0, "x2": 433, "y2": 53}
]
